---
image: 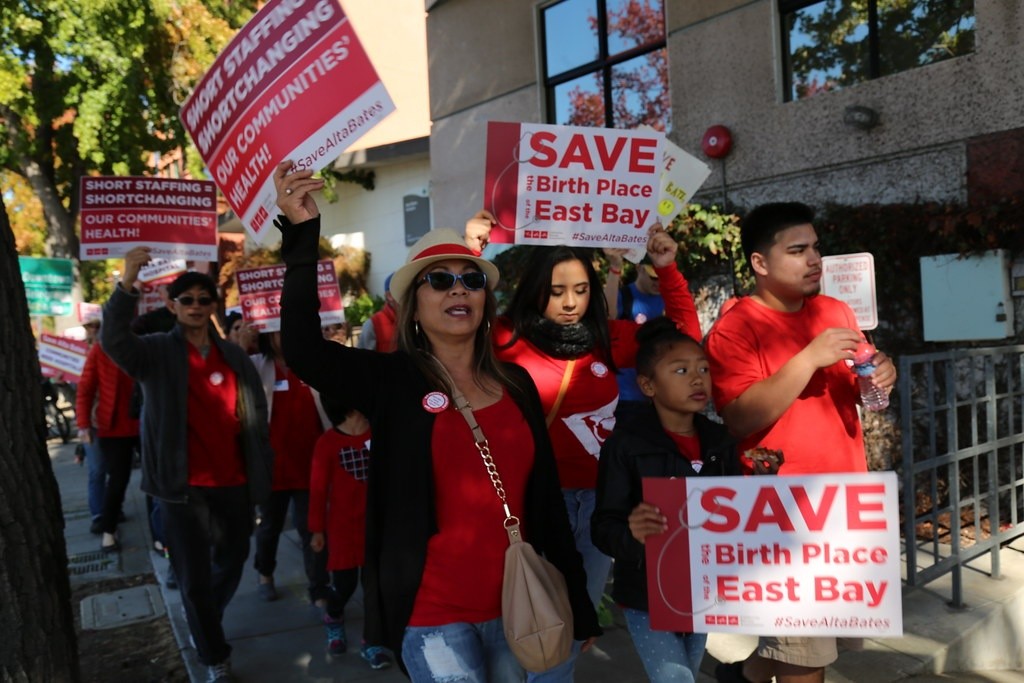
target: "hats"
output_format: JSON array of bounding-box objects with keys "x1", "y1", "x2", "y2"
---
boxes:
[
  {"x1": 388, "y1": 227, "x2": 500, "y2": 305},
  {"x1": 384, "y1": 273, "x2": 394, "y2": 292},
  {"x1": 640, "y1": 252, "x2": 658, "y2": 278},
  {"x1": 82, "y1": 313, "x2": 103, "y2": 326}
]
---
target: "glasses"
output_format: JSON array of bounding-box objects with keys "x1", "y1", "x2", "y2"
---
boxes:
[
  {"x1": 413, "y1": 271, "x2": 489, "y2": 291},
  {"x1": 173, "y1": 296, "x2": 211, "y2": 307},
  {"x1": 321, "y1": 323, "x2": 343, "y2": 331}
]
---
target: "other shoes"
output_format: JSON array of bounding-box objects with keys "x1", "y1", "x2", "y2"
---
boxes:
[
  {"x1": 306, "y1": 588, "x2": 330, "y2": 607},
  {"x1": 101, "y1": 533, "x2": 119, "y2": 554},
  {"x1": 326, "y1": 623, "x2": 345, "y2": 653},
  {"x1": 206, "y1": 658, "x2": 232, "y2": 682},
  {"x1": 360, "y1": 644, "x2": 389, "y2": 669},
  {"x1": 153, "y1": 541, "x2": 164, "y2": 554},
  {"x1": 257, "y1": 577, "x2": 275, "y2": 600}
]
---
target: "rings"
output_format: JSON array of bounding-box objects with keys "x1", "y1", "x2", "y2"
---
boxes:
[{"x1": 286, "y1": 188, "x2": 292, "y2": 195}]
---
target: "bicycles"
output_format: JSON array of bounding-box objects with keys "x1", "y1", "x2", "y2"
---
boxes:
[{"x1": 41, "y1": 382, "x2": 71, "y2": 445}]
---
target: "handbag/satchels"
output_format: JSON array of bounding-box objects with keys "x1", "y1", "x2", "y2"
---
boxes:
[{"x1": 501, "y1": 541, "x2": 571, "y2": 670}]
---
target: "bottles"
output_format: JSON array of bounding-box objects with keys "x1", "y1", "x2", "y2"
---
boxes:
[{"x1": 849, "y1": 337, "x2": 890, "y2": 412}]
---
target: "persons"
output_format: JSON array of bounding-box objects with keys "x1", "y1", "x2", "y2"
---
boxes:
[
  {"x1": 50, "y1": 160, "x2": 783, "y2": 683},
  {"x1": 705, "y1": 201, "x2": 898, "y2": 683}
]
